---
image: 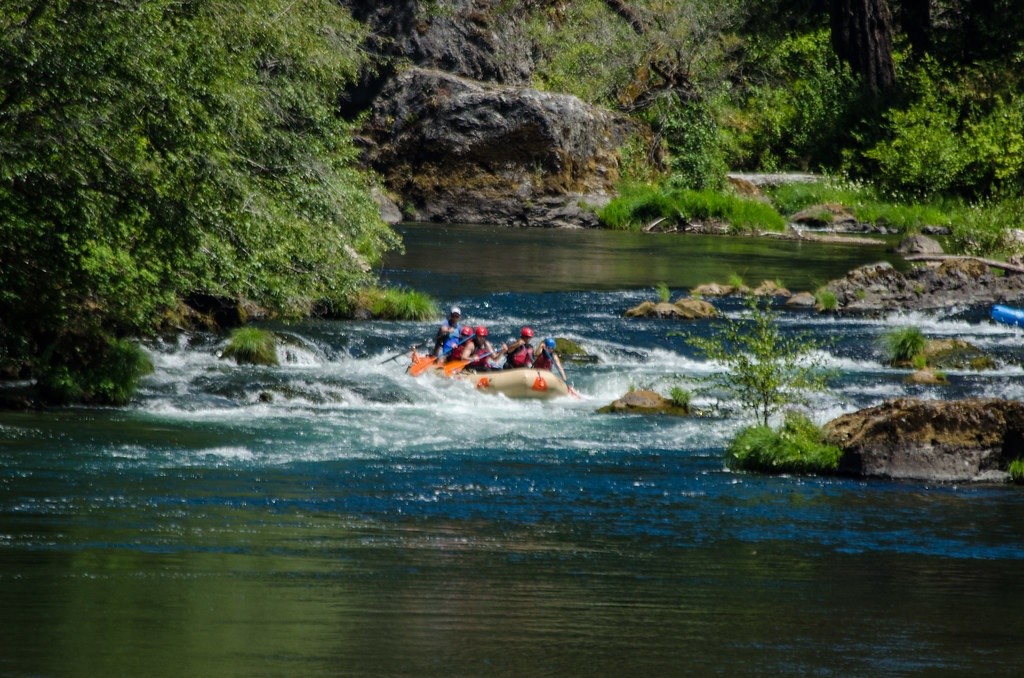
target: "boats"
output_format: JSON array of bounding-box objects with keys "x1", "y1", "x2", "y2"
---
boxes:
[
  {"x1": 408, "y1": 348, "x2": 572, "y2": 403},
  {"x1": 990, "y1": 301, "x2": 1024, "y2": 330}
]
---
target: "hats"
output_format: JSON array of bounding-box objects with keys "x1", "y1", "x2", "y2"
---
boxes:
[{"x1": 450, "y1": 306, "x2": 461, "y2": 315}]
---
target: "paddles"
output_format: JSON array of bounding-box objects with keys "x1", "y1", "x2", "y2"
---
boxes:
[
  {"x1": 443, "y1": 339, "x2": 522, "y2": 380},
  {"x1": 376, "y1": 333, "x2": 445, "y2": 365},
  {"x1": 409, "y1": 332, "x2": 477, "y2": 377},
  {"x1": 540, "y1": 346, "x2": 579, "y2": 398}
]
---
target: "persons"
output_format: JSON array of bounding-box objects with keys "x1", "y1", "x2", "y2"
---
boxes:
[
  {"x1": 463, "y1": 325, "x2": 508, "y2": 375},
  {"x1": 451, "y1": 327, "x2": 473, "y2": 363},
  {"x1": 531, "y1": 338, "x2": 570, "y2": 384},
  {"x1": 435, "y1": 306, "x2": 462, "y2": 363},
  {"x1": 508, "y1": 327, "x2": 536, "y2": 370}
]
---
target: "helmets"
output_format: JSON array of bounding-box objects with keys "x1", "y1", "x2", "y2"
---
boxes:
[
  {"x1": 475, "y1": 326, "x2": 488, "y2": 336},
  {"x1": 522, "y1": 328, "x2": 534, "y2": 337},
  {"x1": 544, "y1": 338, "x2": 557, "y2": 349},
  {"x1": 461, "y1": 326, "x2": 473, "y2": 336}
]
